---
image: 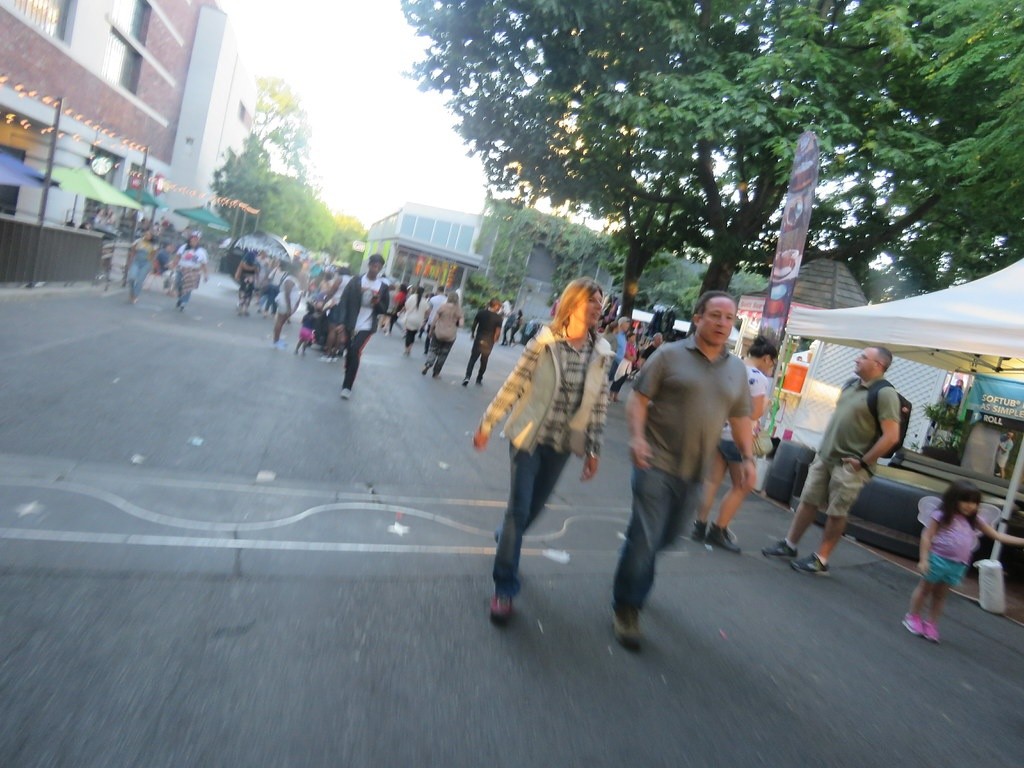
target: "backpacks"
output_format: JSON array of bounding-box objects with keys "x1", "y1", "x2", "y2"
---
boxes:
[{"x1": 841, "y1": 380, "x2": 912, "y2": 460}]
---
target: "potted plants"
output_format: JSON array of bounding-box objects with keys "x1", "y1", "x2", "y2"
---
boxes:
[{"x1": 921, "y1": 401, "x2": 955, "y2": 461}]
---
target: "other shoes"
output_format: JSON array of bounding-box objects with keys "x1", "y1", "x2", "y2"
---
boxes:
[
  {"x1": 432, "y1": 372, "x2": 442, "y2": 380},
  {"x1": 474, "y1": 378, "x2": 482, "y2": 384},
  {"x1": 461, "y1": 377, "x2": 469, "y2": 386},
  {"x1": 690, "y1": 520, "x2": 707, "y2": 542},
  {"x1": 612, "y1": 606, "x2": 640, "y2": 646},
  {"x1": 341, "y1": 388, "x2": 350, "y2": 398},
  {"x1": 705, "y1": 523, "x2": 741, "y2": 555},
  {"x1": 490, "y1": 593, "x2": 511, "y2": 623},
  {"x1": 422, "y1": 362, "x2": 430, "y2": 374}
]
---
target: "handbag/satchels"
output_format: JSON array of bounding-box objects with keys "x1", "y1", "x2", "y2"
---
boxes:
[
  {"x1": 568, "y1": 426, "x2": 585, "y2": 456},
  {"x1": 753, "y1": 430, "x2": 773, "y2": 455}
]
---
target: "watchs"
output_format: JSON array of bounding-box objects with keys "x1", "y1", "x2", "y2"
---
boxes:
[{"x1": 859, "y1": 459, "x2": 867, "y2": 467}]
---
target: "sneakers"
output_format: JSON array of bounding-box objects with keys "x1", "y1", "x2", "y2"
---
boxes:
[
  {"x1": 762, "y1": 538, "x2": 798, "y2": 560},
  {"x1": 902, "y1": 613, "x2": 924, "y2": 636},
  {"x1": 921, "y1": 619, "x2": 939, "y2": 644},
  {"x1": 790, "y1": 552, "x2": 830, "y2": 576}
]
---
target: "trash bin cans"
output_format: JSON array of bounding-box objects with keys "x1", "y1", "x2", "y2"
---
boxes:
[{"x1": 521, "y1": 323, "x2": 541, "y2": 346}]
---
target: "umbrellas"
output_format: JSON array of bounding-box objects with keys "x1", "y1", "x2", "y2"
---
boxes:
[
  {"x1": 174, "y1": 206, "x2": 231, "y2": 232},
  {"x1": 42, "y1": 167, "x2": 141, "y2": 223},
  {"x1": 0, "y1": 152, "x2": 56, "y2": 188},
  {"x1": 127, "y1": 190, "x2": 157, "y2": 206}
]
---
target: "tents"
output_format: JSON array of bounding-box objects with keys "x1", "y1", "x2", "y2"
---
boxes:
[{"x1": 760, "y1": 251, "x2": 1024, "y2": 425}]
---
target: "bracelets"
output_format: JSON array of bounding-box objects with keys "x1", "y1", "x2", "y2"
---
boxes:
[{"x1": 742, "y1": 455, "x2": 753, "y2": 460}]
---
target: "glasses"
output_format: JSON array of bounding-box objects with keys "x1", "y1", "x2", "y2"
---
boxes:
[{"x1": 857, "y1": 354, "x2": 882, "y2": 367}]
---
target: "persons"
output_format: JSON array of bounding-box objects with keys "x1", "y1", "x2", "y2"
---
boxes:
[
  {"x1": 377, "y1": 274, "x2": 525, "y2": 385},
  {"x1": 127, "y1": 217, "x2": 210, "y2": 312},
  {"x1": 235, "y1": 251, "x2": 353, "y2": 360},
  {"x1": 602, "y1": 317, "x2": 663, "y2": 401},
  {"x1": 611, "y1": 290, "x2": 755, "y2": 649},
  {"x1": 79, "y1": 208, "x2": 116, "y2": 229},
  {"x1": 692, "y1": 337, "x2": 776, "y2": 552},
  {"x1": 473, "y1": 276, "x2": 609, "y2": 625},
  {"x1": 995, "y1": 432, "x2": 1014, "y2": 479},
  {"x1": 902, "y1": 480, "x2": 1024, "y2": 643},
  {"x1": 337, "y1": 255, "x2": 388, "y2": 399},
  {"x1": 761, "y1": 346, "x2": 902, "y2": 576}
]
